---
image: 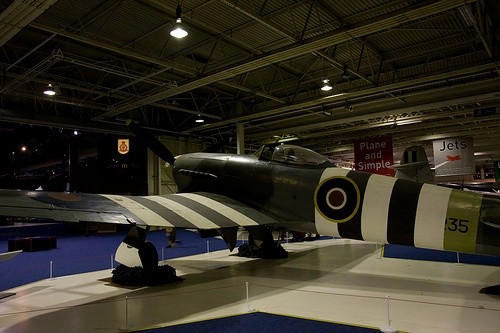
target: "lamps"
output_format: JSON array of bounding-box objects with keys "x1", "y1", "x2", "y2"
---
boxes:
[
  {"x1": 43, "y1": 83, "x2": 56, "y2": 96},
  {"x1": 195, "y1": 114, "x2": 204, "y2": 122},
  {"x1": 321, "y1": 59, "x2": 332, "y2": 91},
  {"x1": 170, "y1": 0, "x2": 188, "y2": 38}
]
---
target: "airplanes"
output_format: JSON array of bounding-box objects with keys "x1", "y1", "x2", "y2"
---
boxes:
[{"x1": 1, "y1": 120, "x2": 500, "y2": 297}]
